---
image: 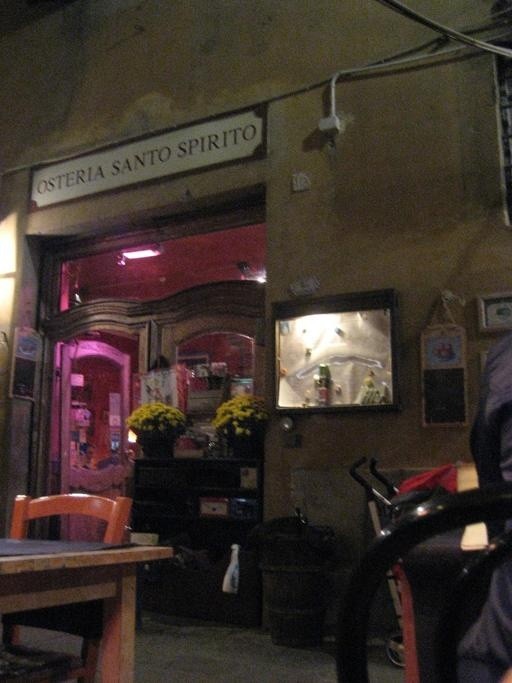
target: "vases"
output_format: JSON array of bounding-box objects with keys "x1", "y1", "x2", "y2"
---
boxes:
[
  {"x1": 233, "y1": 437, "x2": 264, "y2": 458},
  {"x1": 141, "y1": 438, "x2": 175, "y2": 459}
]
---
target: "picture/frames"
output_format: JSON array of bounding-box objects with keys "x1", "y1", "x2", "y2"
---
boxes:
[{"x1": 476, "y1": 292, "x2": 512, "y2": 331}]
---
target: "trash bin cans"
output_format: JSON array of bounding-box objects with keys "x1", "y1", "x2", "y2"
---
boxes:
[{"x1": 258, "y1": 521, "x2": 337, "y2": 649}]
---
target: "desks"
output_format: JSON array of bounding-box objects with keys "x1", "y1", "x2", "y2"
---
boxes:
[{"x1": 0, "y1": 537, "x2": 172, "y2": 683}]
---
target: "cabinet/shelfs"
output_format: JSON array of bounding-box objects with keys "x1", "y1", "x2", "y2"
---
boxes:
[{"x1": 131, "y1": 450, "x2": 261, "y2": 629}]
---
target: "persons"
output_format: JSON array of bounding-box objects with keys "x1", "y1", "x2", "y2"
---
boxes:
[{"x1": 445, "y1": 333, "x2": 511, "y2": 682}]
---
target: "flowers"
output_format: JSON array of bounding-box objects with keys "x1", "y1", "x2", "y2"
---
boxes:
[
  {"x1": 212, "y1": 392, "x2": 271, "y2": 438},
  {"x1": 124, "y1": 400, "x2": 190, "y2": 436}
]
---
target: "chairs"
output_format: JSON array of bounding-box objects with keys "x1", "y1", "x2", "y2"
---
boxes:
[{"x1": 0, "y1": 492, "x2": 135, "y2": 682}]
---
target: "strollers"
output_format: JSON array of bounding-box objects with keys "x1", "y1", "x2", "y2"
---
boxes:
[{"x1": 334, "y1": 331, "x2": 512, "y2": 683}]
